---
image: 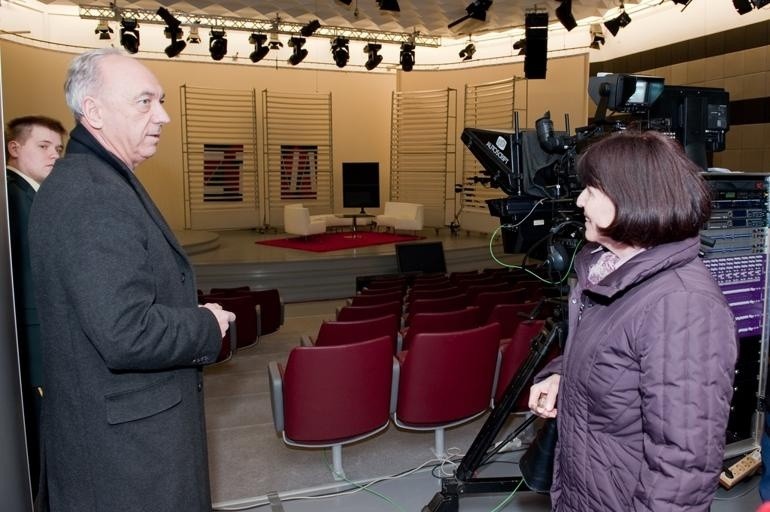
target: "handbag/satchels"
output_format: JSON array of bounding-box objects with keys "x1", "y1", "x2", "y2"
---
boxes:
[{"x1": 518, "y1": 417, "x2": 559, "y2": 495}]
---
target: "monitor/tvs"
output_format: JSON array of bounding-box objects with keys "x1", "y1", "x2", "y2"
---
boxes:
[{"x1": 342, "y1": 161, "x2": 380, "y2": 214}]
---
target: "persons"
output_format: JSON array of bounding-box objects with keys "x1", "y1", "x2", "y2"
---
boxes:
[
  {"x1": 28, "y1": 48, "x2": 236, "y2": 512},
  {"x1": 528, "y1": 131, "x2": 739, "y2": 512},
  {"x1": 4, "y1": 115, "x2": 66, "y2": 512}
]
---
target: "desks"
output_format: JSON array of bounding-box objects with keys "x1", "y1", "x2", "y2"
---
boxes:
[{"x1": 335, "y1": 213, "x2": 376, "y2": 235}]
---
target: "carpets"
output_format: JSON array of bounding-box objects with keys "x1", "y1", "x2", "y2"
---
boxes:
[{"x1": 256, "y1": 229, "x2": 427, "y2": 254}]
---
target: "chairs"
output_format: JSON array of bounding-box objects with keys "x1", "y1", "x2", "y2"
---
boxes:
[
  {"x1": 266, "y1": 262, "x2": 566, "y2": 483},
  {"x1": 198, "y1": 286, "x2": 266, "y2": 366}
]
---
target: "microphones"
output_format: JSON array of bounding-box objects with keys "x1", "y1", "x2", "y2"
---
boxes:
[{"x1": 543, "y1": 259, "x2": 570, "y2": 294}]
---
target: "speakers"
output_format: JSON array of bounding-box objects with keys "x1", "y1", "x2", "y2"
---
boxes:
[{"x1": 524, "y1": 13, "x2": 549, "y2": 79}]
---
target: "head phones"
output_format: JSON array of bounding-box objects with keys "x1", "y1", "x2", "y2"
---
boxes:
[{"x1": 546, "y1": 221, "x2": 586, "y2": 279}]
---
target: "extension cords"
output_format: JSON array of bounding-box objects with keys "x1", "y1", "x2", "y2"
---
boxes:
[
  {"x1": 494, "y1": 437, "x2": 522, "y2": 453},
  {"x1": 719, "y1": 448, "x2": 762, "y2": 490}
]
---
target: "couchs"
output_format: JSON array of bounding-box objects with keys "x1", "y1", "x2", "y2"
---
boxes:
[
  {"x1": 375, "y1": 201, "x2": 426, "y2": 236},
  {"x1": 285, "y1": 203, "x2": 372, "y2": 241}
]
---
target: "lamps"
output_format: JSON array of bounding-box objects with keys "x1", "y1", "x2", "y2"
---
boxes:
[
  {"x1": 375, "y1": 0, "x2": 770, "y2": 81},
  {"x1": 97, "y1": 1, "x2": 417, "y2": 74}
]
